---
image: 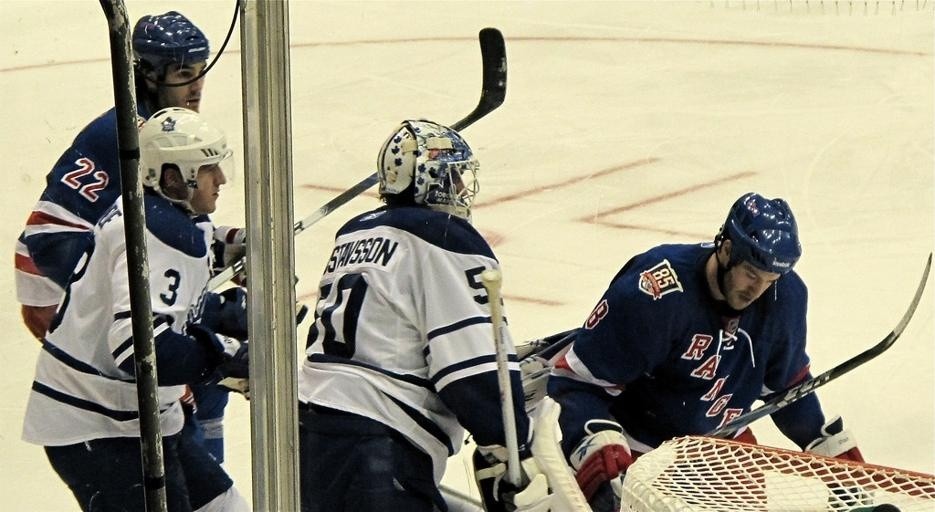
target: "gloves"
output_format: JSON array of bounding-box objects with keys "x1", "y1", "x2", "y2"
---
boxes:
[
  {"x1": 568, "y1": 430, "x2": 633, "y2": 511},
  {"x1": 212, "y1": 226, "x2": 247, "y2": 286},
  {"x1": 472, "y1": 443, "x2": 554, "y2": 512},
  {"x1": 187, "y1": 287, "x2": 249, "y2": 397},
  {"x1": 802, "y1": 414, "x2": 876, "y2": 512}
]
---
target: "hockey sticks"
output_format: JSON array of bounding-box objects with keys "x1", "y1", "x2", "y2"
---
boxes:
[
  {"x1": 701, "y1": 253, "x2": 933, "y2": 439},
  {"x1": 206, "y1": 27, "x2": 507, "y2": 289}
]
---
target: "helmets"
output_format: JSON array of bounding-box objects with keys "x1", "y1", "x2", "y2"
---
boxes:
[
  {"x1": 138, "y1": 106, "x2": 233, "y2": 188},
  {"x1": 132, "y1": 12, "x2": 207, "y2": 68},
  {"x1": 376, "y1": 119, "x2": 474, "y2": 222},
  {"x1": 722, "y1": 192, "x2": 802, "y2": 274}
]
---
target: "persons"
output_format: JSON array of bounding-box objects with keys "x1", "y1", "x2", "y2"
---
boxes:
[
  {"x1": 17, "y1": 102, "x2": 252, "y2": 510},
  {"x1": 13, "y1": 9, "x2": 248, "y2": 465},
  {"x1": 544, "y1": 193, "x2": 905, "y2": 510},
  {"x1": 300, "y1": 113, "x2": 532, "y2": 510}
]
